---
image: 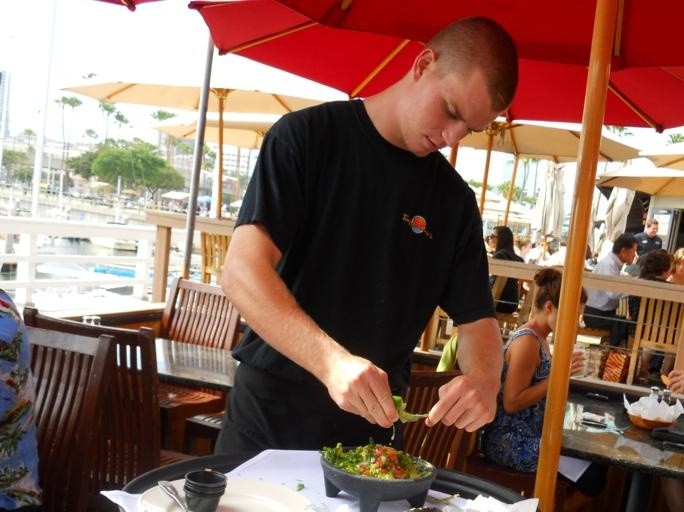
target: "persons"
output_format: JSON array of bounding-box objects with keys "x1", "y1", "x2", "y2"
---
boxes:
[
  {"x1": 211, "y1": 18, "x2": 518, "y2": 461},
  {"x1": 489, "y1": 218, "x2": 684, "y2": 350},
  {"x1": 0, "y1": 286, "x2": 43, "y2": 512},
  {"x1": 476, "y1": 267, "x2": 611, "y2": 512},
  {"x1": 648, "y1": 370, "x2": 684, "y2": 512}
]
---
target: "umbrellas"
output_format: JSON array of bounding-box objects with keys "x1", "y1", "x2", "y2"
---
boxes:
[{"x1": 187, "y1": 1, "x2": 684, "y2": 512}]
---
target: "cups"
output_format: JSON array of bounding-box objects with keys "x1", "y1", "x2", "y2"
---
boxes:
[{"x1": 181, "y1": 469, "x2": 227, "y2": 512}]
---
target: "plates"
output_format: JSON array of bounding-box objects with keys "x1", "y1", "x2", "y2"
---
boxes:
[{"x1": 138, "y1": 477, "x2": 314, "y2": 512}]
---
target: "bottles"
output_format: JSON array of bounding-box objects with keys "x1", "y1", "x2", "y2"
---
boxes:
[
  {"x1": 572, "y1": 342, "x2": 590, "y2": 377},
  {"x1": 648, "y1": 386, "x2": 672, "y2": 407}
]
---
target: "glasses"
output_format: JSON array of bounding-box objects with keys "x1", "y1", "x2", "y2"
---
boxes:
[{"x1": 488, "y1": 234, "x2": 498, "y2": 241}]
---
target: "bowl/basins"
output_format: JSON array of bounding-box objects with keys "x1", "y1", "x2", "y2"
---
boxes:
[
  {"x1": 320, "y1": 447, "x2": 438, "y2": 510},
  {"x1": 625, "y1": 411, "x2": 677, "y2": 429}
]
